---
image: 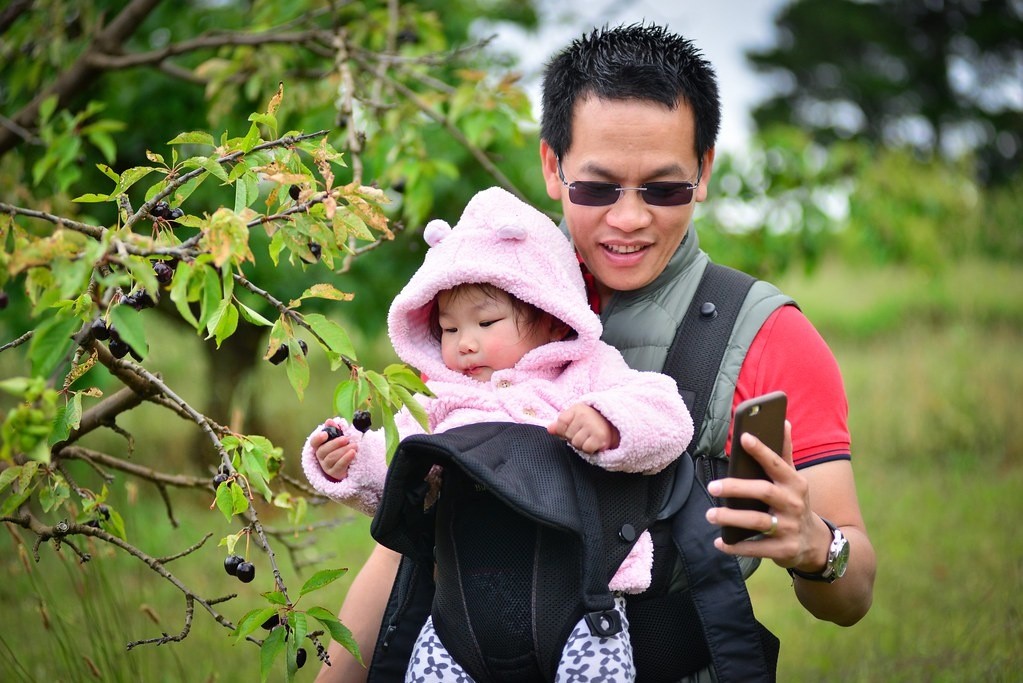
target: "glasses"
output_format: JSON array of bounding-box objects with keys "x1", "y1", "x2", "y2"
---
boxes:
[{"x1": 555, "y1": 153, "x2": 703, "y2": 207}]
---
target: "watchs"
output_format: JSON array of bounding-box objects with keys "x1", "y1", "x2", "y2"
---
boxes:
[{"x1": 786, "y1": 517, "x2": 850, "y2": 583}]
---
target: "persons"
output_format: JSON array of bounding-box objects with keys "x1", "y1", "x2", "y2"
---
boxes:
[
  {"x1": 315, "y1": 19, "x2": 876, "y2": 683},
  {"x1": 302, "y1": 187, "x2": 694, "y2": 683}
]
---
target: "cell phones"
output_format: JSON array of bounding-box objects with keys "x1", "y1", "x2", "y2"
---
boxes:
[{"x1": 720, "y1": 391, "x2": 789, "y2": 543}]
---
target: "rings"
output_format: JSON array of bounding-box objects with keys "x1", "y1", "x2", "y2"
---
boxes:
[{"x1": 763, "y1": 512, "x2": 777, "y2": 535}]
